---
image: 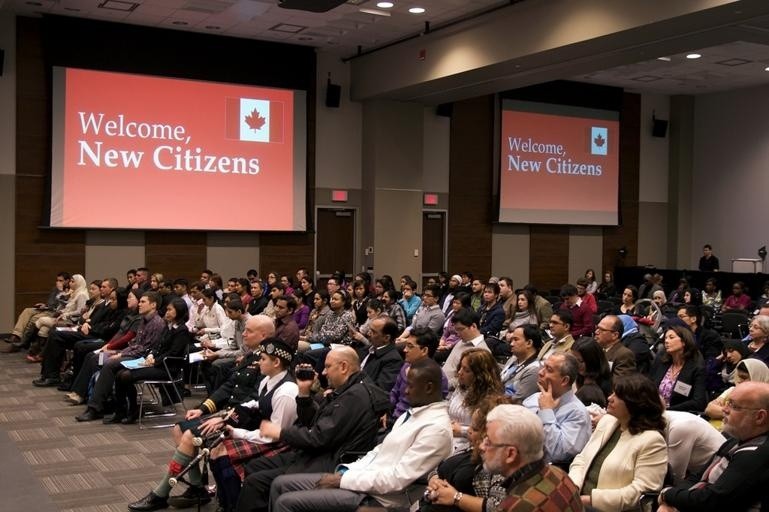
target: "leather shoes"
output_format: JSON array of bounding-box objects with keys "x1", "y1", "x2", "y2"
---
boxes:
[
  {"x1": 129, "y1": 491, "x2": 170, "y2": 511},
  {"x1": 1, "y1": 335, "x2": 20, "y2": 352},
  {"x1": 32, "y1": 374, "x2": 87, "y2": 405},
  {"x1": 75, "y1": 407, "x2": 145, "y2": 424},
  {"x1": 167, "y1": 485, "x2": 211, "y2": 508}
]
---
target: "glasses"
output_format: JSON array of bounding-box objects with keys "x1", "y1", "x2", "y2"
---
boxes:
[
  {"x1": 483, "y1": 435, "x2": 520, "y2": 455},
  {"x1": 594, "y1": 325, "x2": 616, "y2": 332},
  {"x1": 727, "y1": 398, "x2": 760, "y2": 411}
]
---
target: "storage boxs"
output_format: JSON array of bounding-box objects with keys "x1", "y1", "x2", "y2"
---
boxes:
[{"x1": 729, "y1": 257, "x2": 762, "y2": 273}]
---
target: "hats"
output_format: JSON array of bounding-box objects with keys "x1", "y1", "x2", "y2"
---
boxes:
[
  {"x1": 258, "y1": 338, "x2": 294, "y2": 362},
  {"x1": 454, "y1": 274, "x2": 463, "y2": 286}
]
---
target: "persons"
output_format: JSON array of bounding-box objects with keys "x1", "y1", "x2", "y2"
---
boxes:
[
  {"x1": 1, "y1": 268, "x2": 769, "y2": 511},
  {"x1": 698, "y1": 244, "x2": 719, "y2": 272}
]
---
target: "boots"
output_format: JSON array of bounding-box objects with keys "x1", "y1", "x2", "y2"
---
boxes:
[{"x1": 13, "y1": 322, "x2": 47, "y2": 354}]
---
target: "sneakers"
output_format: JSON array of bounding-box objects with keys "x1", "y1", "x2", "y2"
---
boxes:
[{"x1": 27, "y1": 355, "x2": 44, "y2": 362}]
